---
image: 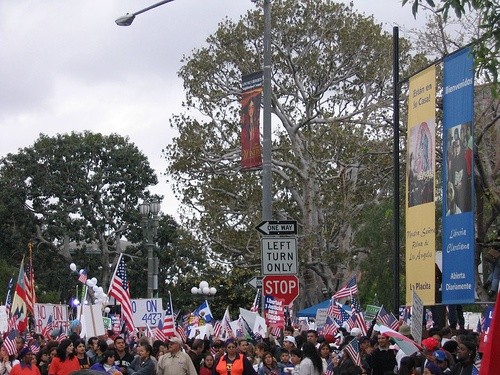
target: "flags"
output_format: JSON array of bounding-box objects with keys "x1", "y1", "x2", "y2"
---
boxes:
[
  {"x1": 3, "y1": 329, "x2": 17, "y2": 356},
  {"x1": 192, "y1": 292, "x2": 262, "y2": 338},
  {"x1": 5, "y1": 258, "x2": 66, "y2": 353},
  {"x1": 108, "y1": 255, "x2": 135, "y2": 332},
  {"x1": 79, "y1": 268, "x2": 87, "y2": 283},
  {"x1": 145, "y1": 296, "x2": 186, "y2": 343},
  {"x1": 320, "y1": 275, "x2": 401, "y2": 337},
  {"x1": 324, "y1": 363, "x2": 334, "y2": 375},
  {"x1": 345, "y1": 338, "x2": 361, "y2": 366}
]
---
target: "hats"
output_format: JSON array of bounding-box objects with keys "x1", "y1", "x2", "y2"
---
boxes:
[
  {"x1": 284, "y1": 336, "x2": 297, "y2": 349},
  {"x1": 359, "y1": 336, "x2": 370, "y2": 342},
  {"x1": 168, "y1": 337, "x2": 183, "y2": 346},
  {"x1": 432, "y1": 350, "x2": 446, "y2": 362}
]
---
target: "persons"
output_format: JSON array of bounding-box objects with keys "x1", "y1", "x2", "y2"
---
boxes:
[
  {"x1": 446, "y1": 123, "x2": 472, "y2": 216},
  {"x1": 0, "y1": 324, "x2": 481, "y2": 375}
]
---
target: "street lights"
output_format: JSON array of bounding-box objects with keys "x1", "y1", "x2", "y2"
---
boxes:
[
  {"x1": 138, "y1": 194, "x2": 162, "y2": 298},
  {"x1": 191, "y1": 280, "x2": 217, "y2": 321},
  {"x1": 116, "y1": 0, "x2": 274, "y2": 322}
]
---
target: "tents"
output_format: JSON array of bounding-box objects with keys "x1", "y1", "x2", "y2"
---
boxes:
[{"x1": 296, "y1": 299, "x2": 351, "y2": 318}]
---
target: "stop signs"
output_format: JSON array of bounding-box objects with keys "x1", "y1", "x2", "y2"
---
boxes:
[{"x1": 262, "y1": 274, "x2": 300, "y2": 307}]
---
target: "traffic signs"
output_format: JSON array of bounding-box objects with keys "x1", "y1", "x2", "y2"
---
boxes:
[
  {"x1": 246, "y1": 275, "x2": 261, "y2": 290},
  {"x1": 254, "y1": 220, "x2": 297, "y2": 235}
]
